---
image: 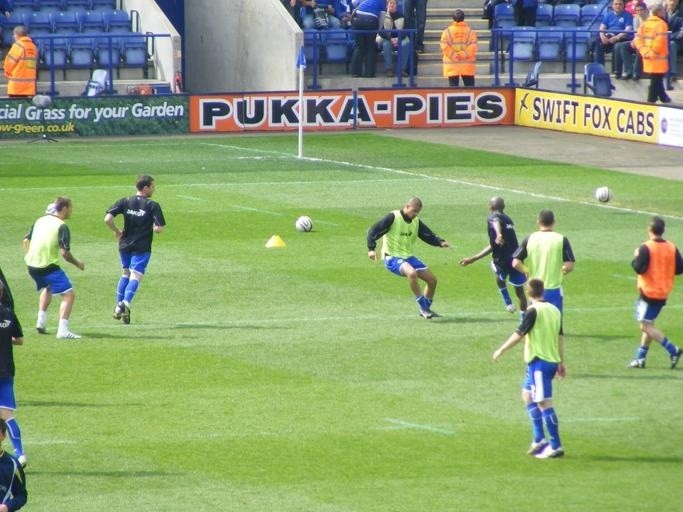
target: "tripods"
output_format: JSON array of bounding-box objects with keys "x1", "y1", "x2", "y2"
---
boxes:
[{"x1": 27, "y1": 106, "x2": 58, "y2": 143}]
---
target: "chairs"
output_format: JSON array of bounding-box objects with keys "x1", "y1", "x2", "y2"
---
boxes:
[
  {"x1": 280, "y1": 0, "x2": 417, "y2": 75},
  {"x1": 0, "y1": 0, "x2": 150, "y2": 78},
  {"x1": 484, "y1": 0, "x2": 683, "y2": 74}
]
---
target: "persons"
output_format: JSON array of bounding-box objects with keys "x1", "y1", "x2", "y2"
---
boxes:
[
  {"x1": 510, "y1": 210, "x2": 575, "y2": 377},
  {"x1": 458, "y1": 195, "x2": 528, "y2": 312},
  {"x1": 366, "y1": 196, "x2": 448, "y2": 319},
  {"x1": 0, "y1": 281, "x2": 27, "y2": 470},
  {"x1": 286, "y1": 1, "x2": 478, "y2": 86},
  {"x1": 0, "y1": 417, "x2": 27, "y2": 511},
  {"x1": 492, "y1": 278, "x2": 566, "y2": 459},
  {"x1": 589, "y1": 0, "x2": 683, "y2": 105},
  {"x1": 630, "y1": 214, "x2": 683, "y2": 368},
  {"x1": 103, "y1": 175, "x2": 167, "y2": 323},
  {"x1": 4, "y1": 25, "x2": 39, "y2": 98},
  {"x1": 23, "y1": 195, "x2": 85, "y2": 339},
  {"x1": 0, "y1": 269, "x2": 14, "y2": 313}
]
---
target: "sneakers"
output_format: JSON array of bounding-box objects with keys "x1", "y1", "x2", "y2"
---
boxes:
[
  {"x1": 35, "y1": 301, "x2": 130, "y2": 339},
  {"x1": 535, "y1": 445, "x2": 563, "y2": 459},
  {"x1": 419, "y1": 304, "x2": 682, "y2": 370},
  {"x1": 528, "y1": 438, "x2": 549, "y2": 455}
]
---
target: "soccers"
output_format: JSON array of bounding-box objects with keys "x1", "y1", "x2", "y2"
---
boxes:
[
  {"x1": 296, "y1": 216, "x2": 312, "y2": 232},
  {"x1": 597, "y1": 186, "x2": 612, "y2": 202}
]
